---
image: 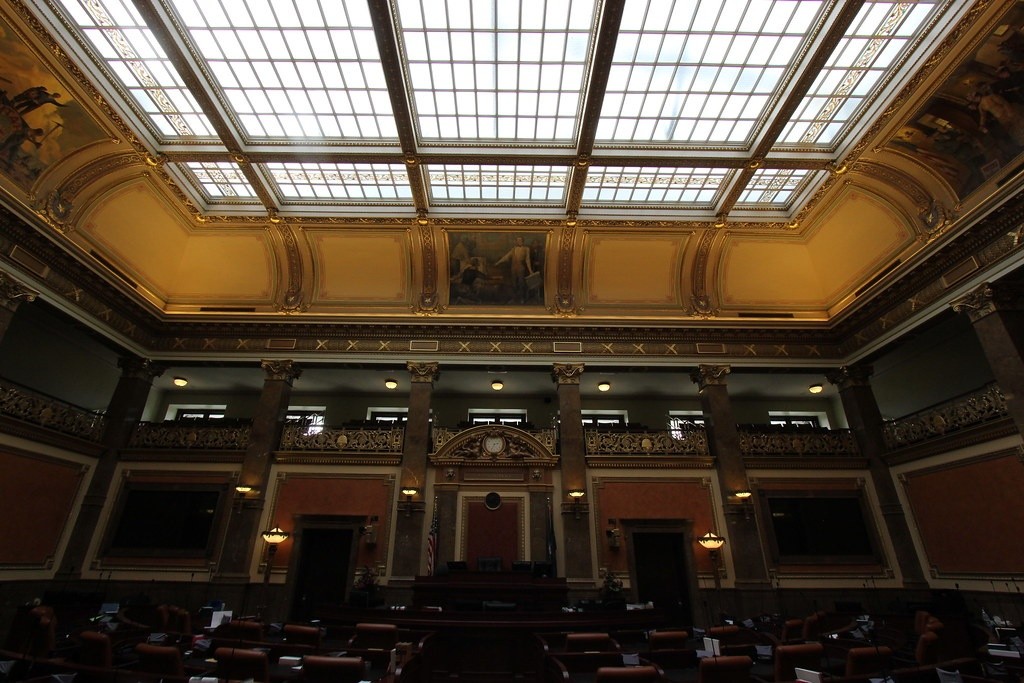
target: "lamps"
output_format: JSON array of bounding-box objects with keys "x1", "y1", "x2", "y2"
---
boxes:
[
  {"x1": 402, "y1": 487, "x2": 419, "y2": 518},
  {"x1": 385, "y1": 380, "x2": 398, "y2": 390},
  {"x1": 174, "y1": 378, "x2": 188, "y2": 387},
  {"x1": 491, "y1": 382, "x2": 503, "y2": 391},
  {"x1": 809, "y1": 385, "x2": 823, "y2": 394},
  {"x1": 234, "y1": 483, "x2": 252, "y2": 514},
  {"x1": 598, "y1": 383, "x2": 611, "y2": 392},
  {"x1": 569, "y1": 489, "x2": 585, "y2": 520},
  {"x1": 734, "y1": 489, "x2": 752, "y2": 522},
  {"x1": 697, "y1": 528, "x2": 728, "y2": 620},
  {"x1": 254, "y1": 524, "x2": 289, "y2": 622}
]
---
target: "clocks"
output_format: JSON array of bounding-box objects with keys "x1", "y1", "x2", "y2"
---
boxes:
[{"x1": 483, "y1": 434, "x2": 506, "y2": 456}]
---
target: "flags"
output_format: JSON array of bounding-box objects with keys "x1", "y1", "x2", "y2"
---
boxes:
[
  {"x1": 426, "y1": 506, "x2": 440, "y2": 575},
  {"x1": 542, "y1": 506, "x2": 557, "y2": 560}
]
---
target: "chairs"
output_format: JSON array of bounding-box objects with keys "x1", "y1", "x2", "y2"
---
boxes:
[
  {"x1": 567, "y1": 601, "x2": 990, "y2": 683},
  {"x1": 21, "y1": 603, "x2": 400, "y2": 683}
]
